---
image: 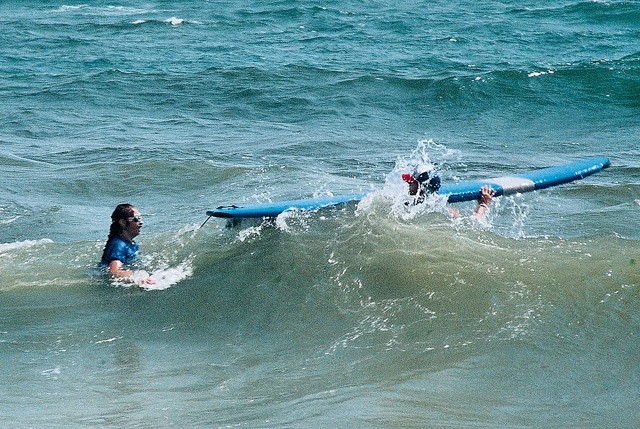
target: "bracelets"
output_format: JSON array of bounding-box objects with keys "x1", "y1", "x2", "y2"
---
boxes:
[{"x1": 479, "y1": 204, "x2": 488, "y2": 209}]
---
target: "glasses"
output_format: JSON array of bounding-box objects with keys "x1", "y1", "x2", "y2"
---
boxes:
[{"x1": 132, "y1": 215, "x2": 143, "y2": 223}]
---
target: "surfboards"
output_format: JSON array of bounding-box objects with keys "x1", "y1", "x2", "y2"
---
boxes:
[{"x1": 205, "y1": 155, "x2": 611, "y2": 229}]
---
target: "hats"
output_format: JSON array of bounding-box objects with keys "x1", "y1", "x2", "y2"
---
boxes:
[{"x1": 402, "y1": 163, "x2": 441, "y2": 193}]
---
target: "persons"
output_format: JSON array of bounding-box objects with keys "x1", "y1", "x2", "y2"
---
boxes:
[
  {"x1": 100, "y1": 203, "x2": 155, "y2": 285},
  {"x1": 405, "y1": 162, "x2": 496, "y2": 222}
]
